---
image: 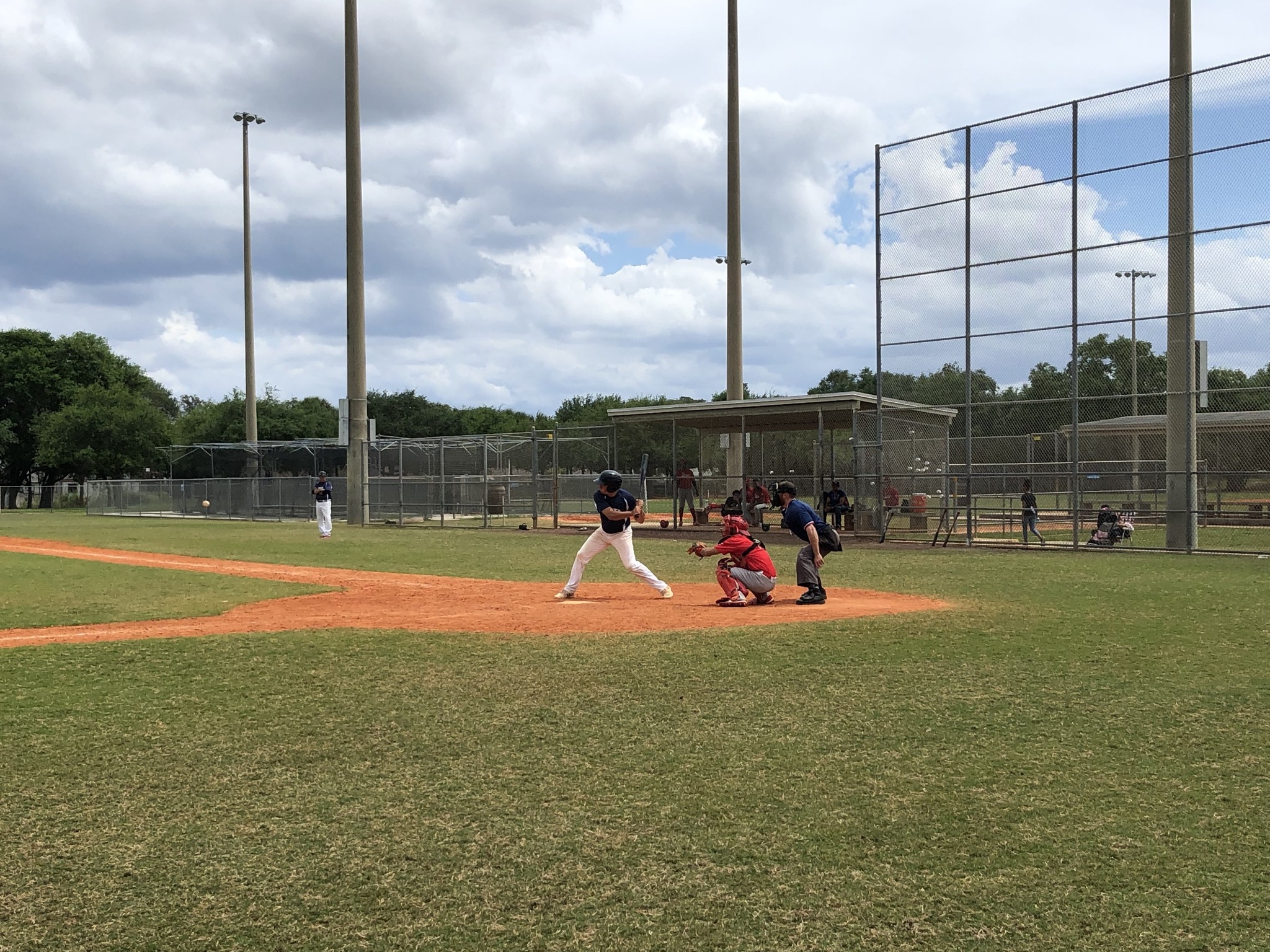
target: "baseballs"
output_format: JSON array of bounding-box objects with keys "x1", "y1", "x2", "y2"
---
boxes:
[{"x1": 202, "y1": 500, "x2": 210, "y2": 507}]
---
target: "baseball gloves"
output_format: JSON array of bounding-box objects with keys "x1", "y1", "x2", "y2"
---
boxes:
[{"x1": 684, "y1": 541, "x2": 708, "y2": 562}]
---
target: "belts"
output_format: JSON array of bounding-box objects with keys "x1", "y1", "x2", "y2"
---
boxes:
[
  {"x1": 763, "y1": 571, "x2": 771, "y2": 579},
  {"x1": 317, "y1": 500, "x2": 327, "y2": 502}
]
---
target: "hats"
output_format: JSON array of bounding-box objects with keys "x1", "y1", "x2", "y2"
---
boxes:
[
  {"x1": 832, "y1": 482, "x2": 839, "y2": 487},
  {"x1": 319, "y1": 471, "x2": 326, "y2": 476},
  {"x1": 730, "y1": 516, "x2": 751, "y2": 536},
  {"x1": 679, "y1": 458, "x2": 689, "y2": 465},
  {"x1": 772, "y1": 480, "x2": 796, "y2": 496}
]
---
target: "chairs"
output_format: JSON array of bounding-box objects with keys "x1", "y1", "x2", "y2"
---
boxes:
[{"x1": 1118, "y1": 512, "x2": 1137, "y2": 544}]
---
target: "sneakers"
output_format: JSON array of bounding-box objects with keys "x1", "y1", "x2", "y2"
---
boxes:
[
  {"x1": 720, "y1": 599, "x2": 747, "y2": 606},
  {"x1": 554, "y1": 589, "x2": 574, "y2": 599},
  {"x1": 757, "y1": 595, "x2": 773, "y2": 604},
  {"x1": 662, "y1": 586, "x2": 673, "y2": 598}
]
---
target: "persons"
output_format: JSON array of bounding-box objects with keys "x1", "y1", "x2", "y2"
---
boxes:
[
  {"x1": 1021, "y1": 479, "x2": 1046, "y2": 546},
  {"x1": 826, "y1": 481, "x2": 849, "y2": 530},
  {"x1": 1092, "y1": 504, "x2": 1118, "y2": 536},
  {"x1": 883, "y1": 478, "x2": 899, "y2": 505},
  {"x1": 312, "y1": 471, "x2": 333, "y2": 537},
  {"x1": 676, "y1": 459, "x2": 700, "y2": 526},
  {"x1": 688, "y1": 514, "x2": 777, "y2": 607},
  {"x1": 767, "y1": 481, "x2": 843, "y2": 604},
  {"x1": 721, "y1": 478, "x2": 769, "y2": 526},
  {"x1": 554, "y1": 470, "x2": 673, "y2": 598}
]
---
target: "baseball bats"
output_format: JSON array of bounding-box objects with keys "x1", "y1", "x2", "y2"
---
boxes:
[
  {"x1": 879, "y1": 508, "x2": 894, "y2": 543},
  {"x1": 635, "y1": 453, "x2": 649, "y2": 518},
  {"x1": 932, "y1": 509, "x2": 947, "y2": 546},
  {"x1": 942, "y1": 511, "x2": 960, "y2": 547}
]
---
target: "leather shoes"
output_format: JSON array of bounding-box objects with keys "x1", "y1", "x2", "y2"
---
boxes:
[{"x1": 796, "y1": 594, "x2": 825, "y2": 604}]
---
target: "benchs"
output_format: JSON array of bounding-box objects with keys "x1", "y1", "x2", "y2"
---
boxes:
[
  {"x1": 687, "y1": 506, "x2": 936, "y2": 530},
  {"x1": 1079, "y1": 499, "x2": 1270, "y2": 518}
]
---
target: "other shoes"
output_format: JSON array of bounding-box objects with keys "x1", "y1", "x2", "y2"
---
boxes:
[
  {"x1": 678, "y1": 522, "x2": 683, "y2": 528},
  {"x1": 324, "y1": 535, "x2": 330, "y2": 538},
  {"x1": 1041, "y1": 538, "x2": 1046, "y2": 547},
  {"x1": 1023, "y1": 543, "x2": 1028, "y2": 545},
  {"x1": 836, "y1": 527, "x2": 842, "y2": 532},
  {"x1": 693, "y1": 522, "x2": 700, "y2": 526},
  {"x1": 320, "y1": 536, "x2": 325, "y2": 538}
]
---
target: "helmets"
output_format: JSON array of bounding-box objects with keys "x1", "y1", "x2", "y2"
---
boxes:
[{"x1": 593, "y1": 470, "x2": 622, "y2": 492}]
